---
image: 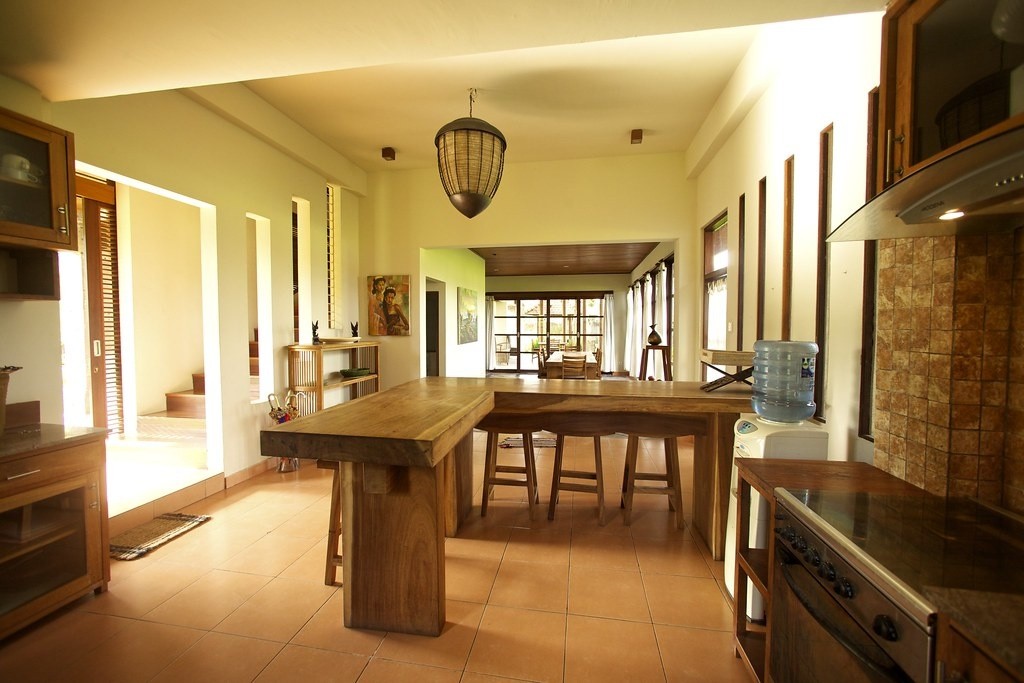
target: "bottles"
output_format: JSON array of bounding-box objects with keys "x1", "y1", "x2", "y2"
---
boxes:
[{"x1": 751, "y1": 341, "x2": 819, "y2": 422}]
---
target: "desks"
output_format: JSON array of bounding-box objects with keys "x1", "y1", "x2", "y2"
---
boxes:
[
  {"x1": 537, "y1": 341, "x2": 565, "y2": 351},
  {"x1": 544, "y1": 350, "x2": 598, "y2": 379}
]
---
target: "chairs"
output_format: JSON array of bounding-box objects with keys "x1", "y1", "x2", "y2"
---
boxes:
[
  {"x1": 531, "y1": 339, "x2": 540, "y2": 363},
  {"x1": 536, "y1": 346, "x2": 602, "y2": 379}
]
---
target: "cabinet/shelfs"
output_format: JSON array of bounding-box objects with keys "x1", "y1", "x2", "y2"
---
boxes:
[
  {"x1": 729, "y1": 456, "x2": 932, "y2": 683},
  {"x1": 286, "y1": 340, "x2": 380, "y2": 417},
  {"x1": 0, "y1": 105, "x2": 77, "y2": 253},
  {"x1": 882, "y1": 0, "x2": 1024, "y2": 188},
  {"x1": 0, "y1": 423, "x2": 114, "y2": 632}
]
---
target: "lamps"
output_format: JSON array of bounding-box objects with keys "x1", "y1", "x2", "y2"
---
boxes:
[{"x1": 433, "y1": 88, "x2": 507, "y2": 221}]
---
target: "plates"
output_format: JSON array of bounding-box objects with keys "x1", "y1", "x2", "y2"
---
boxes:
[{"x1": 319, "y1": 337, "x2": 362, "y2": 344}]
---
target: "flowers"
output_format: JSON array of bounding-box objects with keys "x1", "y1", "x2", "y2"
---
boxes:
[{"x1": 269, "y1": 405, "x2": 300, "y2": 424}]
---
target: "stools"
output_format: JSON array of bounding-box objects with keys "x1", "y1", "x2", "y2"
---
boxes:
[{"x1": 312, "y1": 425, "x2": 684, "y2": 587}]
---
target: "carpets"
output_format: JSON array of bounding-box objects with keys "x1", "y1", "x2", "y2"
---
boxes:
[{"x1": 106, "y1": 511, "x2": 216, "y2": 561}]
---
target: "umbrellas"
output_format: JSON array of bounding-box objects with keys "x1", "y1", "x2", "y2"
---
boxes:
[{"x1": 268, "y1": 390, "x2": 306, "y2": 473}]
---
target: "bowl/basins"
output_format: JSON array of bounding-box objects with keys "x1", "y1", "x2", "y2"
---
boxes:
[{"x1": 3, "y1": 155, "x2": 31, "y2": 172}]
